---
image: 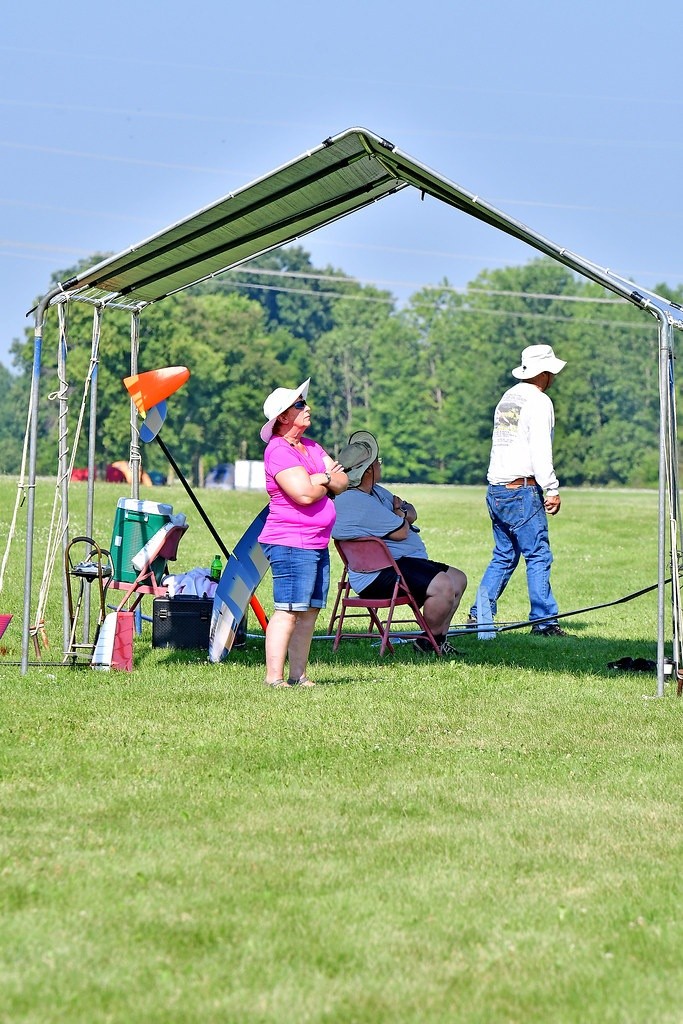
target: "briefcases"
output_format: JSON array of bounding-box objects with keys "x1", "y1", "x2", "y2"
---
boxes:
[{"x1": 152, "y1": 590, "x2": 215, "y2": 650}]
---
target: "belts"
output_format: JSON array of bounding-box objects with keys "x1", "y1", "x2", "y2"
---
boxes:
[{"x1": 511, "y1": 478, "x2": 536, "y2": 485}]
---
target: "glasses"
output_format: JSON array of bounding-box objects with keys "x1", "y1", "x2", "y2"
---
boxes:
[
  {"x1": 374, "y1": 457, "x2": 383, "y2": 465},
  {"x1": 287, "y1": 400, "x2": 307, "y2": 408}
]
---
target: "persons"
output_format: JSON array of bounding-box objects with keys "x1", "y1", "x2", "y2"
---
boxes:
[
  {"x1": 257, "y1": 376, "x2": 349, "y2": 690},
  {"x1": 464, "y1": 343, "x2": 575, "y2": 638},
  {"x1": 330, "y1": 431, "x2": 467, "y2": 658}
]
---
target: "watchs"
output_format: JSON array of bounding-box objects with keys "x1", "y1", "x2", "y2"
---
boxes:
[{"x1": 397, "y1": 507, "x2": 407, "y2": 518}]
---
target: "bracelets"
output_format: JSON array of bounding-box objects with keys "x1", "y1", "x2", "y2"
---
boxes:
[{"x1": 325, "y1": 473, "x2": 331, "y2": 482}]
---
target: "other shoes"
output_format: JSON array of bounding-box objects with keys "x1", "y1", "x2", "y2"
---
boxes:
[
  {"x1": 287, "y1": 676, "x2": 315, "y2": 688},
  {"x1": 435, "y1": 641, "x2": 461, "y2": 656},
  {"x1": 413, "y1": 632, "x2": 433, "y2": 652},
  {"x1": 263, "y1": 677, "x2": 292, "y2": 688}
]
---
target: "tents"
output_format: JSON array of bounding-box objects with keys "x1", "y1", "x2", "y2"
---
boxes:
[{"x1": 0, "y1": 126, "x2": 683, "y2": 698}]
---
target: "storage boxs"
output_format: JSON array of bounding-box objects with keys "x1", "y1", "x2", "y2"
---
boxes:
[
  {"x1": 107, "y1": 497, "x2": 173, "y2": 588},
  {"x1": 152, "y1": 598, "x2": 248, "y2": 651}
]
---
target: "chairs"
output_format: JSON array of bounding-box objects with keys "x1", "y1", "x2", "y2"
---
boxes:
[
  {"x1": 326, "y1": 536, "x2": 443, "y2": 657},
  {"x1": 103, "y1": 523, "x2": 190, "y2": 634}
]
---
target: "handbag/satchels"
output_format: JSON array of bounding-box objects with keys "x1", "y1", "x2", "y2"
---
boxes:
[{"x1": 89, "y1": 612, "x2": 134, "y2": 672}]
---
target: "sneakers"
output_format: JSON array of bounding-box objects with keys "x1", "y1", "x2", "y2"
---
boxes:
[
  {"x1": 466, "y1": 614, "x2": 478, "y2": 629},
  {"x1": 531, "y1": 623, "x2": 566, "y2": 636}
]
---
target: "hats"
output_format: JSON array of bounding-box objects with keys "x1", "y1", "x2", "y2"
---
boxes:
[
  {"x1": 512, "y1": 344, "x2": 567, "y2": 380},
  {"x1": 334, "y1": 430, "x2": 379, "y2": 487},
  {"x1": 260, "y1": 376, "x2": 311, "y2": 444}
]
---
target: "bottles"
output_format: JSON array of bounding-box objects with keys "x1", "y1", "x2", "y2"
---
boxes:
[{"x1": 211, "y1": 555, "x2": 223, "y2": 580}]
